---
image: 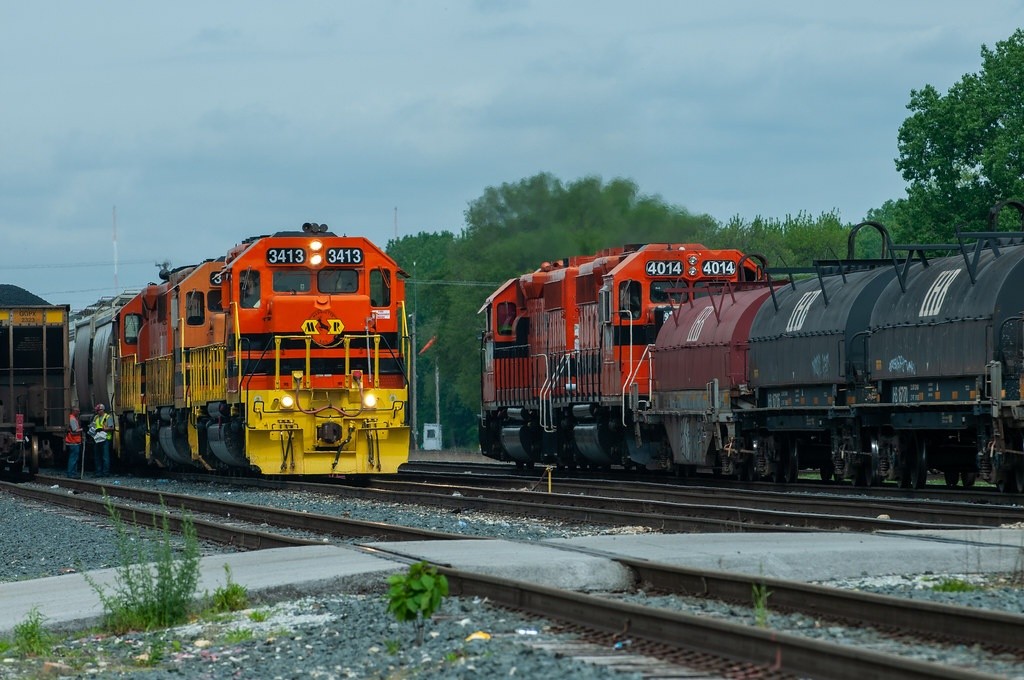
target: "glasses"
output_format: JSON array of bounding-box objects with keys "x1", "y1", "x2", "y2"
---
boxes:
[{"x1": 73, "y1": 410, "x2": 81, "y2": 411}]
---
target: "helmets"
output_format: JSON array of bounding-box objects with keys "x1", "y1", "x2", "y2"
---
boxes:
[{"x1": 95, "y1": 403, "x2": 105, "y2": 411}]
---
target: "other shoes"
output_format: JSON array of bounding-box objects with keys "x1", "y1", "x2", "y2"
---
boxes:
[
  {"x1": 95, "y1": 473, "x2": 109, "y2": 477},
  {"x1": 68, "y1": 475, "x2": 81, "y2": 479}
]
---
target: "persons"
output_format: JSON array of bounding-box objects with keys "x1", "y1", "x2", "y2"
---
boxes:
[
  {"x1": 319, "y1": 269, "x2": 335, "y2": 292},
  {"x1": 65, "y1": 406, "x2": 83, "y2": 479},
  {"x1": 92, "y1": 404, "x2": 114, "y2": 478}
]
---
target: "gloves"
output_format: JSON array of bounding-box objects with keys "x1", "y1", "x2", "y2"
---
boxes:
[{"x1": 95, "y1": 428, "x2": 102, "y2": 432}]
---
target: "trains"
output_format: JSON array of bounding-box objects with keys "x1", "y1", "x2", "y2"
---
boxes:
[
  {"x1": 477, "y1": 217, "x2": 1023, "y2": 497},
  {"x1": 68, "y1": 221, "x2": 414, "y2": 490},
  {"x1": 0, "y1": 284, "x2": 71, "y2": 482}
]
